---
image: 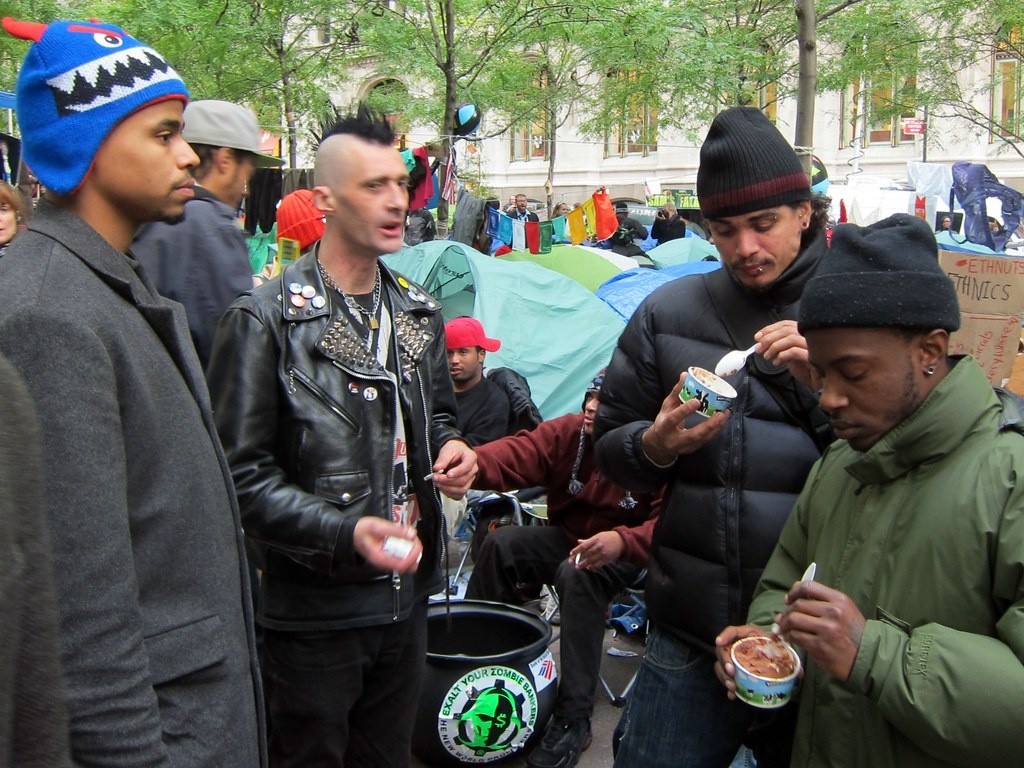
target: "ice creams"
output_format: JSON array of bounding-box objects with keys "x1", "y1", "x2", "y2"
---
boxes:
[{"x1": 734, "y1": 640, "x2": 794, "y2": 679}]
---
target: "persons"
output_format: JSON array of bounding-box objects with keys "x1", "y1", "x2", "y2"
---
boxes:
[
  {"x1": 486, "y1": 195, "x2": 687, "y2": 257},
  {"x1": 989, "y1": 220, "x2": 1023, "y2": 251},
  {"x1": 470, "y1": 365, "x2": 666, "y2": 768},
  {"x1": 440, "y1": 316, "x2": 509, "y2": 457},
  {"x1": 208, "y1": 102, "x2": 480, "y2": 768},
  {"x1": 0, "y1": 24, "x2": 288, "y2": 768},
  {"x1": 593, "y1": 106, "x2": 830, "y2": 768},
  {"x1": 935, "y1": 216, "x2": 957, "y2": 235},
  {"x1": 713, "y1": 214, "x2": 1023, "y2": 768}
]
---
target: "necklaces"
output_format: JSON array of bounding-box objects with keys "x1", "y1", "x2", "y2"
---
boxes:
[{"x1": 316, "y1": 259, "x2": 383, "y2": 330}]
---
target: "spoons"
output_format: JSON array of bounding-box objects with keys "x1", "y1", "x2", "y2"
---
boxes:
[{"x1": 714, "y1": 346, "x2": 755, "y2": 378}]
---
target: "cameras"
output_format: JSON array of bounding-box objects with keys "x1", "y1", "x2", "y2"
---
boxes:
[{"x1": 658, "y1": 209, "x2": 669, "y2": 218}]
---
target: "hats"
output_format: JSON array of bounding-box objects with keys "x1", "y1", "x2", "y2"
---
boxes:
[
  {"x1": 1, "y1": 16, "x2": 192, "y2": 203},
  {"x1": 616, "y1": 202, "x2": 629, "y2": 214},
  {"x1": 795, "y1": 212, "x2": 962, "y2": 338},
  {"x1": 273, "y1": 188, "x2": 327, "y2": 252},
  {"x1": 565, "y1": 365, "x2": 638, "y2": 510},
  {"x1": 444, "y1": 316, "x2": 501, "y2": 352},
  {"x1": 696, "y1": 106, "x2": 812, "y2": 219},
  {"x1": 180, "y1": 99, "x2": 286, "y2": 169}
]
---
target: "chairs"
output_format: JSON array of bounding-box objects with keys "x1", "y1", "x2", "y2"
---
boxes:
[{"x1": 440, "y1": 487, "x2": 648, "y2": 707}]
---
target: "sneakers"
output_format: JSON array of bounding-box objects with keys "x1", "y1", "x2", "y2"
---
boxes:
[{"x1": 523, "y1": 711, "x2": 595, "y2": 768}]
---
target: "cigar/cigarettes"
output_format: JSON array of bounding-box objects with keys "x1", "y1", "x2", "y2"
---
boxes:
[
  {"x1": 383, "y1": 536, "x2": 424, "y2": 566},
  {"x1": 423, "y1": 470, "x2": 445, "y2": 483},
  {"x1": 574, "y1": 551, "x2": 583, "y2": 567}
]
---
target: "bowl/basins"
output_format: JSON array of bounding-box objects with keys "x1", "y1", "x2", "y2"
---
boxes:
[
  {"x1": 678, "y1": 366, "x2": 738, "y2": 419},
  {"x1": 730, "y1": 636, "x2": 801, "y2": 710}
]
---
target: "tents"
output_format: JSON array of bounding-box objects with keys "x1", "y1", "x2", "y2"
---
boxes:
[
  {"x1": 254, "y1": 218, "x2": 729, "y2": 424},
  {"x1": 828, "y1": 160, "x2": 1024, "y2": 255}
]
